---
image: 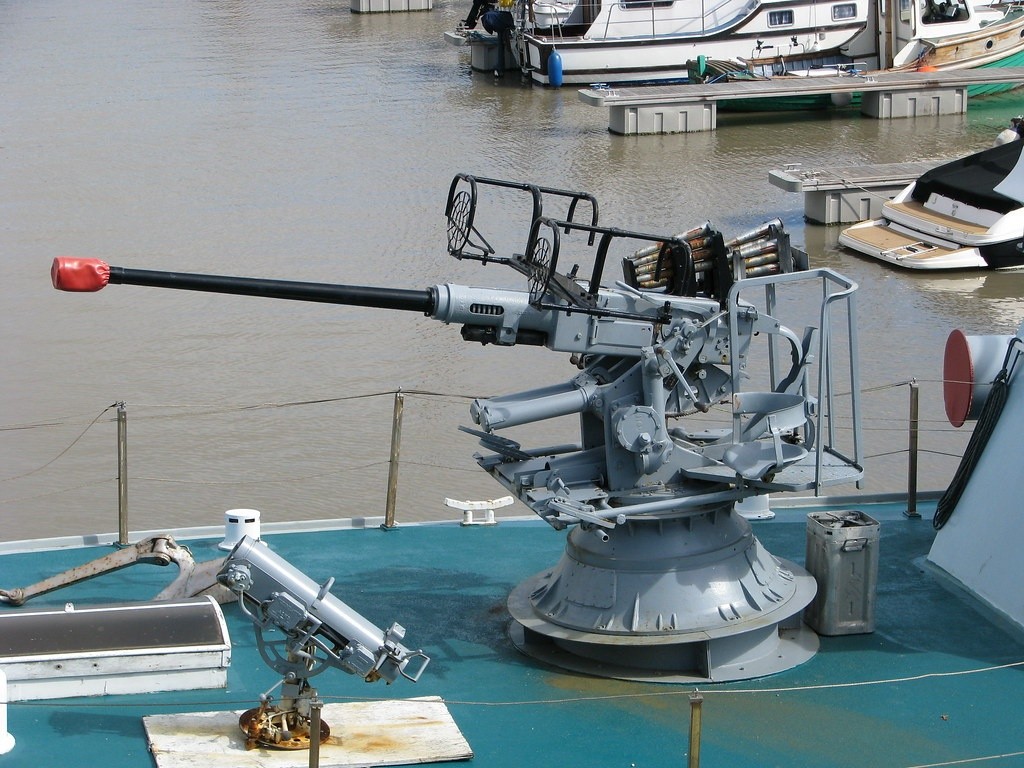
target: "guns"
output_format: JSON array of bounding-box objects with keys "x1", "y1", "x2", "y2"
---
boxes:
[{"x1": 48, "y1": 172, "x2": 868, "y2": 368}]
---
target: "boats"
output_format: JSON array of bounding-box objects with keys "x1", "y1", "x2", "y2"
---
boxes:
[
  {"x1": 452, "y1": 0, "x2": 1024, "y2": 114},
  {"x1": 837, "y1": 113, "x2": 1024, "y2": 274}
]
